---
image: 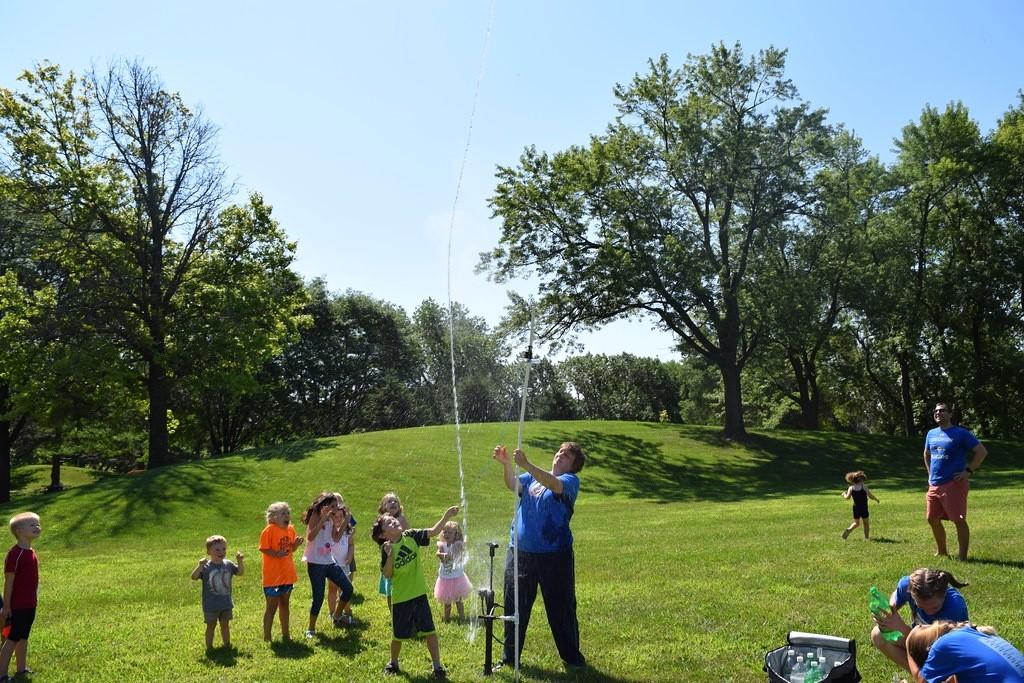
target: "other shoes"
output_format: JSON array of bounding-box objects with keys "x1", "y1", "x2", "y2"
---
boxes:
[
  {"x1": 562, "y1": 661, "x2": 588, "y2": 670},
  {"x1": 344, "y1": 610, "x2": 356, "y2": 621},
  {"x1": 841, "y1": 527, "x2": 850, "y2": 541},
  {"x1": 385, "y1": 661, "x2": 399, "y2": 674},
  {"x1": 430, "y1": 669, "x2": 446, "y2": 681},
  {"x1": 332, "y1": 613, "x2": 354, "y2": 625},
  {"x1": 304, "y1": 631, "x2": 315, "y2": 639},
  {"x1": 492, "y1": 661, "x2": 522, "y2": 673}
]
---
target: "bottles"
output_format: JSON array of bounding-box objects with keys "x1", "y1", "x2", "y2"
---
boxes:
[
  {"x1": 870, "y1": 588, "x2": 903, "y2": 640},
  {"x1": 784, "y1": 650, "x2": 840, "y2": 683}
]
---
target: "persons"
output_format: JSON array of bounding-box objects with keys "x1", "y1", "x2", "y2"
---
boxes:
[
  {"x1": 433, "y1": 520, "x2": 474, "y2": 624},
  {"x1": 372, "y1": 504, "x2": 460, "y2": 678},
  {"x1": 258, "y1": 502, "x2": 303, "y2": 644},
  {"x1": 923, "y1": 402, "x2": 988, "y2": 561},
  {"x1": 191, "y1": 535, "x2": 244, "y2": 649},
  {"x1": 299, "y1": 492, "x2": 357, "y2": 639},
  {"x1": 492, "y1": 441, "x2": 597, "y2": 671},
  {"x1": 904, "y1": 620, "x2": 1024, "y2": 683},
  {"x1": 377, "y1": 492, "x2": 412, "y2": 613},
  {"x1": 838, "y1": 468, "x2": 883, "y2": 540},
  {"x1": 870, "y1": 567, "x2": 969, "y2": 683},
  {"x1": 0, "y1": 511, "x2": 42, "y2": 683}
]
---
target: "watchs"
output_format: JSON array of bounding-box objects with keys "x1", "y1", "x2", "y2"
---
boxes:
[{"x1": 966, "y1": 466, "x2": 973, "y2": 473}]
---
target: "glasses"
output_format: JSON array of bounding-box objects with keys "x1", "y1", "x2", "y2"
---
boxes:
[{"x1": 933, "y1": 408, "x2": 950, "y2": 415}]
